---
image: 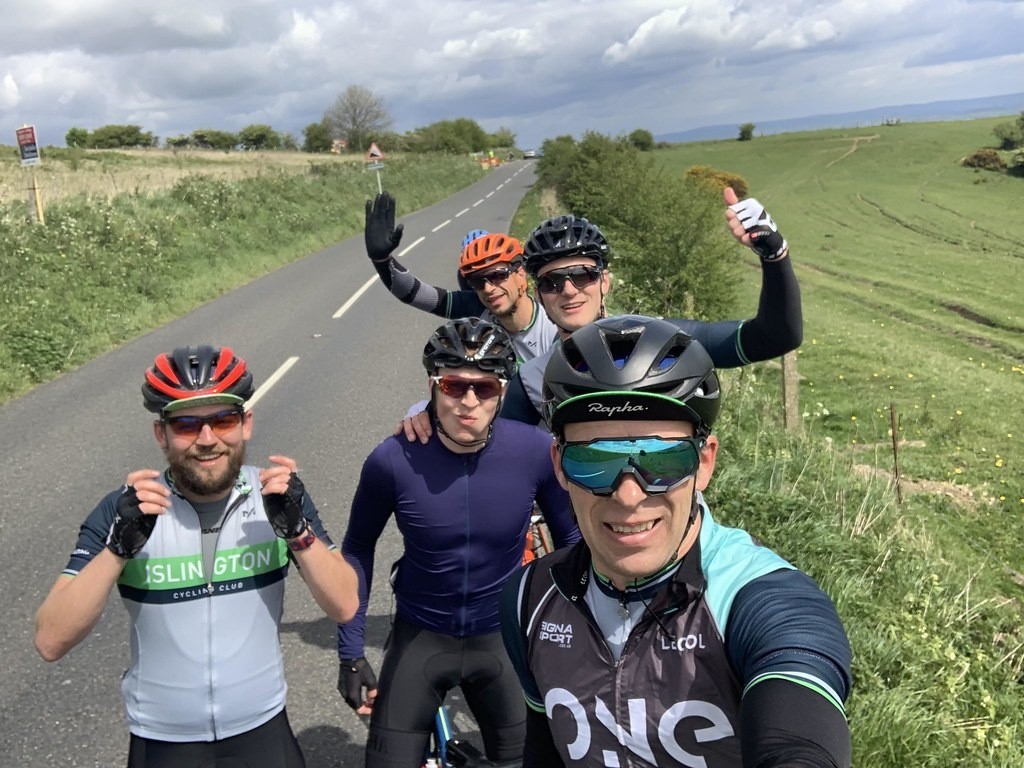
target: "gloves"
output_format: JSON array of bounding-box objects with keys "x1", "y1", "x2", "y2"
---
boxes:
[{"x1": 365, "y1": 191, "x2": 404, "y2": 259}]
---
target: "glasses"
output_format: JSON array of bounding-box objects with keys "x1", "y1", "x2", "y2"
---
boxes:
[
  {"x1": 534, "y1": 264, "x2": 603, "y2": 294},
  {"x1": 467, "y1": 260, "x2": 521, "y2": 290},
  {"x1": 160, "y1": 407, "x2": 244, "y2": 435},
  {"x1": 558, "y1": 435, "x2": 707, "y2": 497},
  {"x1": 429, "y1": 375, "x2": 509, "y2": 400}
]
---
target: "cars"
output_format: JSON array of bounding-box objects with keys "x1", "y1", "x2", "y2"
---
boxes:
[{"x1": 524, "y1": 149, "x2": 537, "y2": 158}]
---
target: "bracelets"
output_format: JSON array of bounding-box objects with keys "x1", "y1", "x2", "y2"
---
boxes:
[{"x1": 286, "y1": 521, "x2": 316, "y2": 552}]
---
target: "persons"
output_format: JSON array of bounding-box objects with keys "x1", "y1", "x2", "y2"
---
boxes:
[
  {"x1": 363, "y1": 185, "x2": 805, "y2": 564},
  {"x1": 496, "y1": 314, "x2": 855, "y2": 768},
  {"x1": 31, "y1": 342, "x2": 361, "y2": 768},
  {"x1": 335, "y1": 316, "x2": 585, "y2": 768},
  {"x1": 506, "y1": 150, "x2": 514, "y2": 162}
]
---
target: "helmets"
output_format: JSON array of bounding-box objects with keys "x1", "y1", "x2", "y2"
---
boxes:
[
  {"x1": 459, "y1": 233, "x2": 524, "y2": 278},
  {"x1": 520, "y1": 215, "x2": 611, "y2": 280},
  {"x1": 461, "y1": 229, "x2": 489, "y2": 252},
  {"x1": 541, "y1": 315, "x2": 721, "y2": 432},
  {"x1": 422, "y1": 317, "x2": 517, "y2": 380},
  {"x1": 141, "y1": 343, "x2": 255, "y2": 418}
]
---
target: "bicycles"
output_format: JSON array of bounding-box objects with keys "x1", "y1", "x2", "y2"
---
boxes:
[
  {"x1": 522, "y1": 499, "x2": 552, "y2": 568},
  {"x1": 421, "y1": 705, "x2": 499, "y2": 768}
]
